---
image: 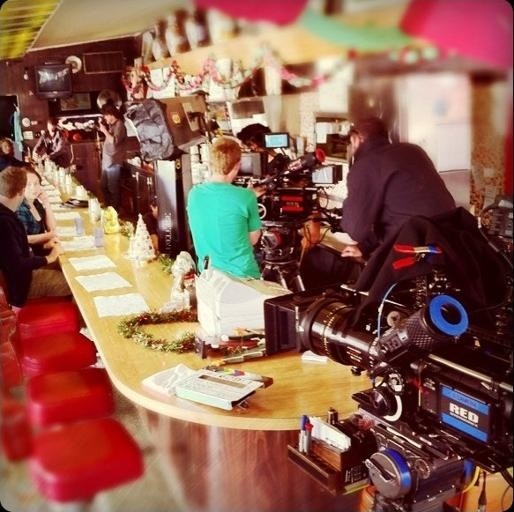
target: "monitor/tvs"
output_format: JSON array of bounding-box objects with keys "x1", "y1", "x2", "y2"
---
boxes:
[{"x1": 33, "y1": 66, "x2": 74, "y2": 99}]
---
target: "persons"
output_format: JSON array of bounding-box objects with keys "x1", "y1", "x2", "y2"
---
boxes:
[
  {"x1": 99, "y1": 102, "x2": 126, "y2": 212},
  {"x1": 186, "y1": 137, "x2": 262, "y2": 280},
  {"x1": 16, "y1": 165, "x2": 58, "y2": 244},
  {"x1": 340, "y1": 115, "x2": 455, "y2": 254},
  {"x1": 1, "y1": 166, "x2": 72, "y2": 306},
  {"x1": 33, "y1": 115, "x2": 71, "y2": 168},
  {"x1": 0, "y1": 137, "x2": 31, "y2": 171}
]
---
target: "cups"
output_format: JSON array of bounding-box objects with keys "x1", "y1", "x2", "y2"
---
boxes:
[
  {"x1": 74, "y1": 217, "x2": 84, "y2": 236},
  {"x1": 95, "y1": 228, "x2": 105, "y2": 247}
]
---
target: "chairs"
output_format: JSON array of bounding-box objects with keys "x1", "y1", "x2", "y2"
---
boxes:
[{"x1": 0, "y1": 280, "x2": 147, "y2": 512}]
